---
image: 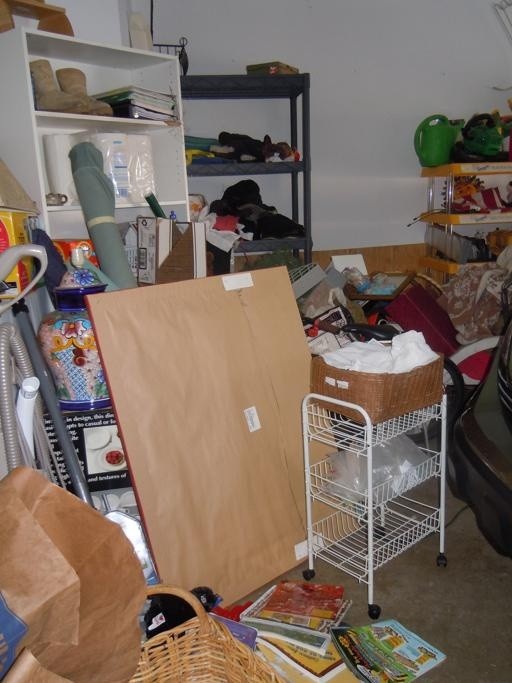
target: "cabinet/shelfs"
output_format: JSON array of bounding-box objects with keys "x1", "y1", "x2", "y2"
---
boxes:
[
  {"x1": 303, "y1": 389, "x2": 447, "y2": 619},
  {"x1": 1, "y1": 28, "x2": 190, "y2": 240},
  {"x1": 419, "y1": 160, "x2": 512, "y2": 285},
  {"x1": 180, "y1": 73, "x2": 313, "y2": 268}
]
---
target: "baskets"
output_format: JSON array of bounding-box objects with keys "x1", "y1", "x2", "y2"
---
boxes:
[
  {"x1": 125, "y1": 586, "x2": 293, "y2": 682},
  {"x1": 309, "y1": 342, "x2": 444, "y2": 425}
]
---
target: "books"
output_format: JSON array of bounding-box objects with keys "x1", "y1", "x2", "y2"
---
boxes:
[
  {"x1": 238, "y1": 578, "x2": 446, "y2": 683},
  {"x1": 93, "y1": 86, "x2": 178, "y2": 122}
]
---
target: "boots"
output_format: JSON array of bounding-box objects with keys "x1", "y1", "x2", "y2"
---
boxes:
[
  {"x1": 31, "y1": 59, "x2": 84, "y2": 112},
  {"x1": 56, "y1": 67, "x2": 113, "y2": 116}
]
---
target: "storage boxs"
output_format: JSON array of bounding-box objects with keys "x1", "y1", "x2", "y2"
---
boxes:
[{"x1": 384, "y1": 283, "x2": 458, "y2": 355}]
